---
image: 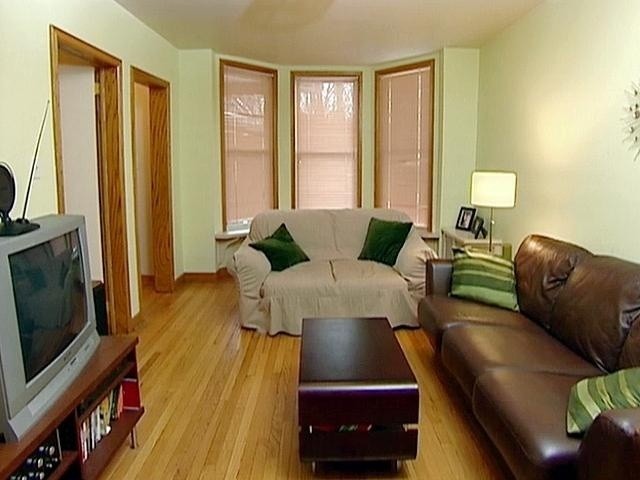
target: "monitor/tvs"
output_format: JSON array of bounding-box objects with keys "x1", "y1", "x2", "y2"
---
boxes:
[{"x1": 0, "y1": 212, "x2": 103, "y2": 444}]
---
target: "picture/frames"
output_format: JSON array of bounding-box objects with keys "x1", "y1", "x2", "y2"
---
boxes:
[
  {"x1": 473, "y1": 217, "x2": 487, "y2": 239},
  {"x1": 456, "y1": 205, "x2": 477, "y2": 230}
]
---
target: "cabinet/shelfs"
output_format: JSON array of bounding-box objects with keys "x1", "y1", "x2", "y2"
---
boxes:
[
  {"x1": 441, "y1": 230, "x2": 503, "y2": 260},
  {"x1": 1, "y1": 335, "x2": 144, "y2": 480}
]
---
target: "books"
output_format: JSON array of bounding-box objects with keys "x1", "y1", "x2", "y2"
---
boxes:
[{"x1": 79, "y1": 383, "x2": 126, "y2": 465}]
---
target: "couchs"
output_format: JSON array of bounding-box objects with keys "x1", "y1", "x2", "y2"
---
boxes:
[
  {"x1": 419, "y1": 235, "x2": 640, "y2": 479},
  {"x1": 227, "y1": 207, "x2": 439, "y2": 337}
]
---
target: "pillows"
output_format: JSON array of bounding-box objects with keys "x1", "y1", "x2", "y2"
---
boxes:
[
  {"x1": 566, "y1": 368, "x2": 640, "y2": 437},
  {"x1": 358, "y1": 217, "x2": 413, "y2": 266},
  {"x1": 248, "y1": 222, "x2": 313, "y2": 272},
  {"x1": 452, "y1": 245, "x2": 521, "y2": 313}
]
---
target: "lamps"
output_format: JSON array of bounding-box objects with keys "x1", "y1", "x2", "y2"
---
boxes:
[{"x1": 470, "y1": 170, "x2": 516, "y2": 252}]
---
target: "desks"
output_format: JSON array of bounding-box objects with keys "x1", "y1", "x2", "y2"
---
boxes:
[{"x1": 299, "y1": 317, "x2": 419, "y2": 475}]
